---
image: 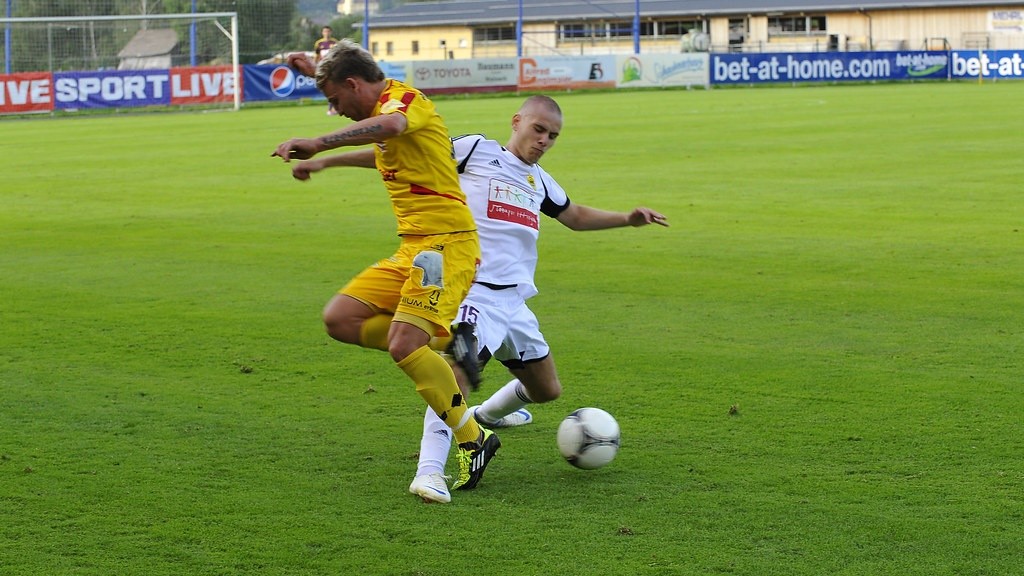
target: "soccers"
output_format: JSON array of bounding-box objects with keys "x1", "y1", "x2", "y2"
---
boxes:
[{"x1": 556, "y1": 407, "x2": 621, "y2": 470}]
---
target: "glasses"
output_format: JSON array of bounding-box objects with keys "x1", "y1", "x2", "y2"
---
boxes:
[{"x1": 327, "y1": 97, "x2": 340, "y2": 115}]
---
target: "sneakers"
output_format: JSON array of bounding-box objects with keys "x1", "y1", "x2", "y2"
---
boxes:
[
  {"x1": 469, "y1": 405, "x2": 533, "y2": 426},
  {"x1": 451, "y1": 428, "x2": 501, "y2": 491},
  {"x1": 445, "y1": 323, "x2": 480, "y2": 392},
  {"x1": 409, "y1": 473, "x2": 451, "y2": 503}
]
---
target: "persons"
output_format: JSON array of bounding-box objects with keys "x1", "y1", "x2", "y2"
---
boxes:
[
  {"x1": 313, "y1": 26, "x2": 344, "y2": 116},
  {"x1": 275, "y1": 44, "x2": 502, "y2": 492},
  {"x1": 406, "y1": 95, "x2": 672, "y2": 503}
]
